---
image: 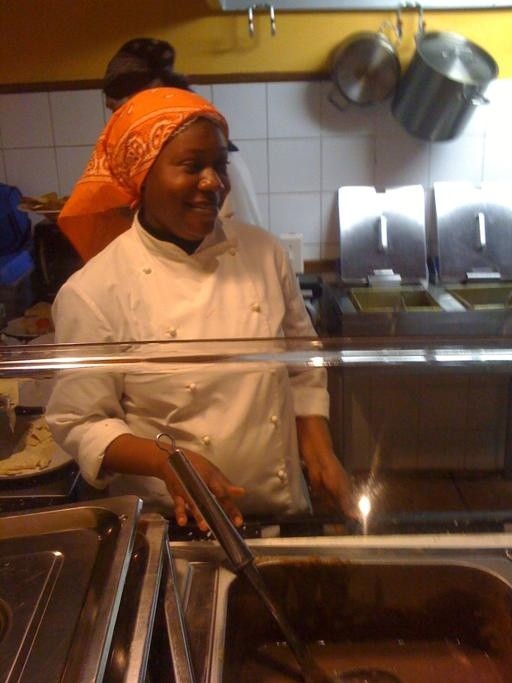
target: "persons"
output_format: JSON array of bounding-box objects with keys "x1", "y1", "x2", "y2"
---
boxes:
[
  {"x1": 101, "y1": 38, "x2": 265, "y2": 231},
  {"x1": 42, "y1": 87, "x2": 379, "y2": 535}
]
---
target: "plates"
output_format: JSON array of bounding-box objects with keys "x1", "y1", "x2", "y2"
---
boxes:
[
  {"x1": 0, "y1": 410, "x2": 74, "y2": 481},
  {"x1": 16, "y1": 199, "x2": 64, "y2": 215},
  {"x1": 22, "y1": 300, "x2": 53, "y2": 318},
  {"x1": 23, "y1": 331, "x2": 56, "y2": 345},
  {"x1": 0, "y1": 316, "x2": 49, "y2": 337}
]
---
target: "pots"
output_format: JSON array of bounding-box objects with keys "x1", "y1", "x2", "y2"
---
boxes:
[{"x1": 325, "y1": 18, "x2": 404, "y2": 109}]
